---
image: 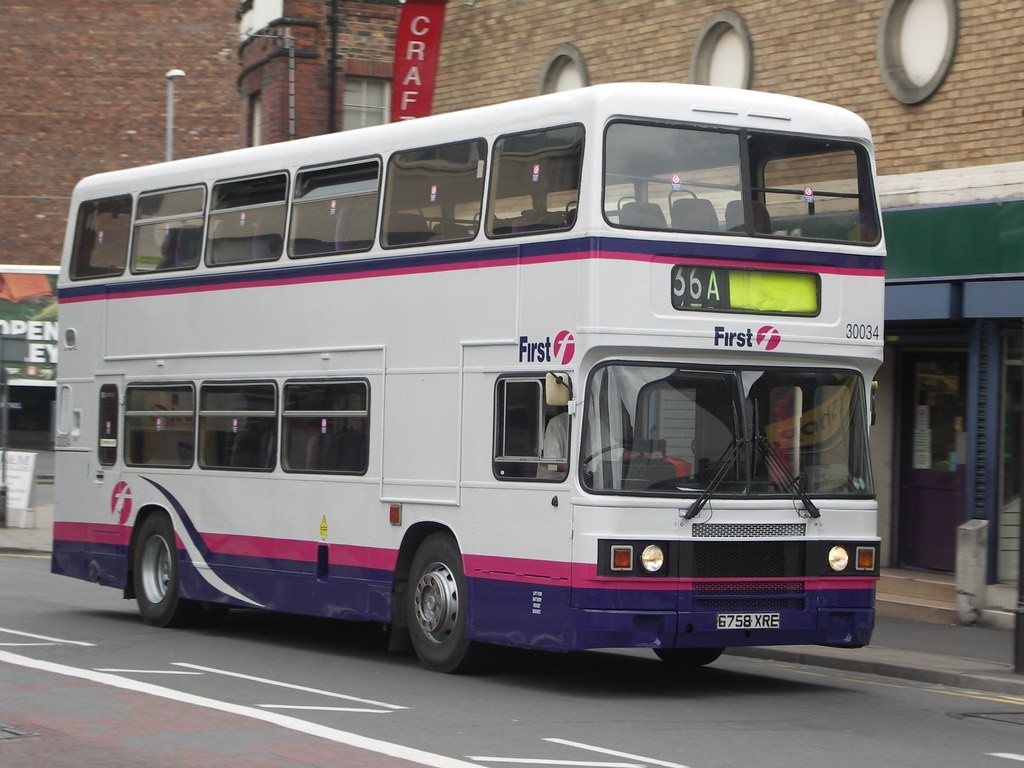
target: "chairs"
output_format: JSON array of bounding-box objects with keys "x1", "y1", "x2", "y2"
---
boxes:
[
  {"x1": 668, "y1": 190, "x2": 718, "y2": 232},
  {"x1": 176, "y1": 189, "x2": 577, "y2": 267},
  {"x1": 616, "y1": 196, "x2": 668, "y2": 228},
  {"x1": 231, "y1": 420, "x2": 367, "y2": 472},
  {"x1": 726, "y1": 199, "x2": 771, "y2": 233}
]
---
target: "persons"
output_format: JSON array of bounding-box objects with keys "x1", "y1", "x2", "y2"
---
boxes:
[{"x1": 544, "y1": 381, "x2": 664, "y2": 476}]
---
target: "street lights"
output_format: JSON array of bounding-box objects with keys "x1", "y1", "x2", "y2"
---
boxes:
[{"x1": 164, "y1": 68, "x2": 187, "y2": 163}]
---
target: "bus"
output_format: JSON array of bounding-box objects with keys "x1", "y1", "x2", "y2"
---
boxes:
[{"x1": 43, "y1": 81, "x2": 890, "y2": 676}]
